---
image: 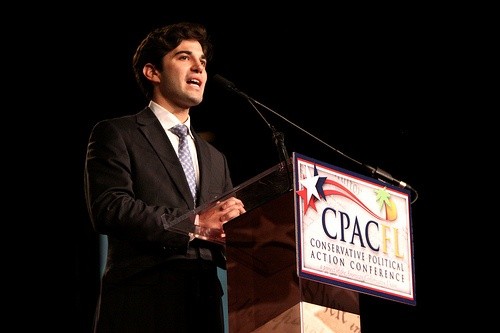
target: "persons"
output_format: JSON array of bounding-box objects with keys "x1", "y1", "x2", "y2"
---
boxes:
[{"x1": 82, "y1": 24, "x2": 246, "y2": 332}]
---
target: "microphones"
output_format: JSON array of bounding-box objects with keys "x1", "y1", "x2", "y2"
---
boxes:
[{"x1": 214, "y1": 74, "x2": 239, "y2": 93}]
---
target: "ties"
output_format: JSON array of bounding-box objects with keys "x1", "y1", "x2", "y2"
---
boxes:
[{"x1": 168, "y1": 124, "x2": 198, "y2": 210}]
